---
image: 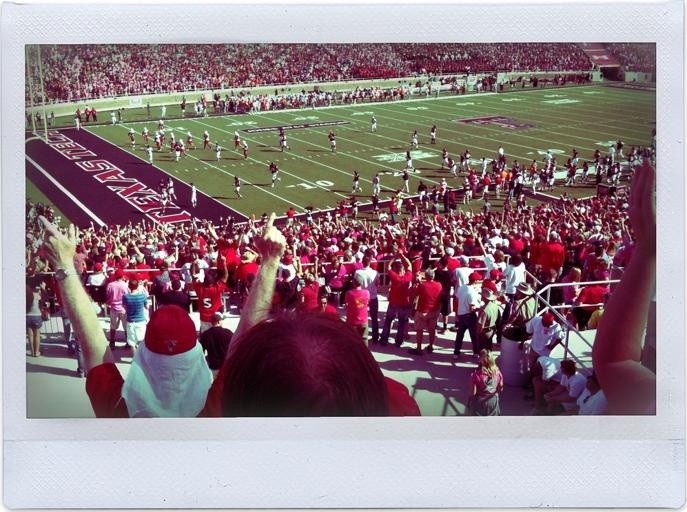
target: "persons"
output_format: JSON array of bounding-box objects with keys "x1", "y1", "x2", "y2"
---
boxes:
[
  {"x1": 28, "y1": 125, "x2": 655, "y2": 418},
  {"x1": 26, "y1": 43, "x2": 654, "y2": 130}
]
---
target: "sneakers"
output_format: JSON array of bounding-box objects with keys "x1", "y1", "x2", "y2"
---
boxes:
[{"x1": 523, "y1": 392, "x2": 535, "y2": 400}]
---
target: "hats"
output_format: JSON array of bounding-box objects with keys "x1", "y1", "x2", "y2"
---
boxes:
[
  {"x1": 460, "y1": 255, "x2": 469, "y2": 267},
  {"x1": 515, "y1": 282, "x2": 534, "y2": 296},
  {"x1": 212, "y1": 312, "x2": 226, "y2": 321},
  {"x1": 543, "y1": 313, "x2": 554, "y2": 325},
  {"x1": 468, "y1": 272, "x2": 482, "y2": 282},
  {"x1": 445, "y1": 247, "x2": 455, "y2": 256},
  {"x1": 478, "y1": 288, "x2": 498, "y2": 301},
  {"x1": 146, "y1": 303, "x2": 197, "y2": 355}
]
